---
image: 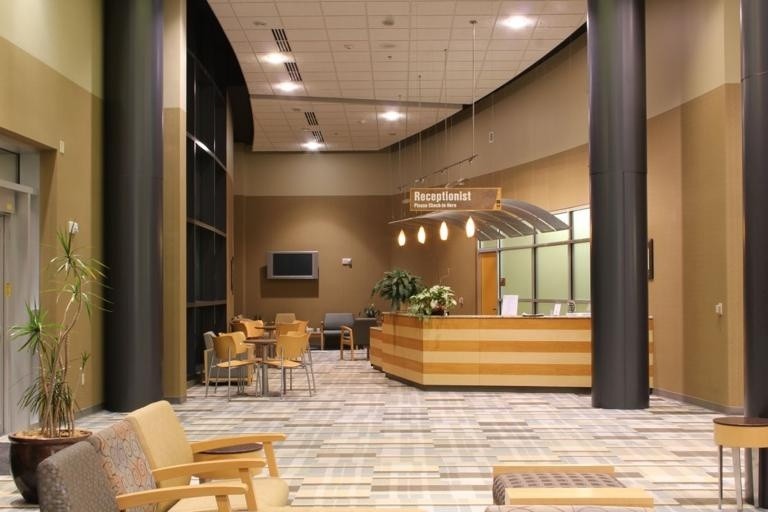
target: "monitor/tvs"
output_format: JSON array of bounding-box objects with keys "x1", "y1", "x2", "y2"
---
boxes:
[{"x1": 266, "y1": 251, "x2": 319, "y2": 280}]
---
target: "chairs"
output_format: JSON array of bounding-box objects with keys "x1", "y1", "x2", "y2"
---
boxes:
[
  {"x1": 34, "y1": 398, "x2": 290, "y2": 511},
  {"x1": 321, "y1": 313, "x2": 376, "y2": 363},
  {"x1": 200, "y1": 312, "x2": 316, "y2": 401}
]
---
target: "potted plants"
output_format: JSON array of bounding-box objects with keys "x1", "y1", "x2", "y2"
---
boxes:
[
  {"x1": 8, "y1": 216, "x2": 114, "y2": 506},
  {"x1": 359, "y1": 271, "x2": 458, "y2": 317}
]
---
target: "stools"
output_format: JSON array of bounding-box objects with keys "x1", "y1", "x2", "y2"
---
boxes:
[
  {"x1": 713, "y1": 415, "x2": 768, "y2": 511},
  {"x1": 483, "y1": 463, "x2": 651, "y2": 503}
]
